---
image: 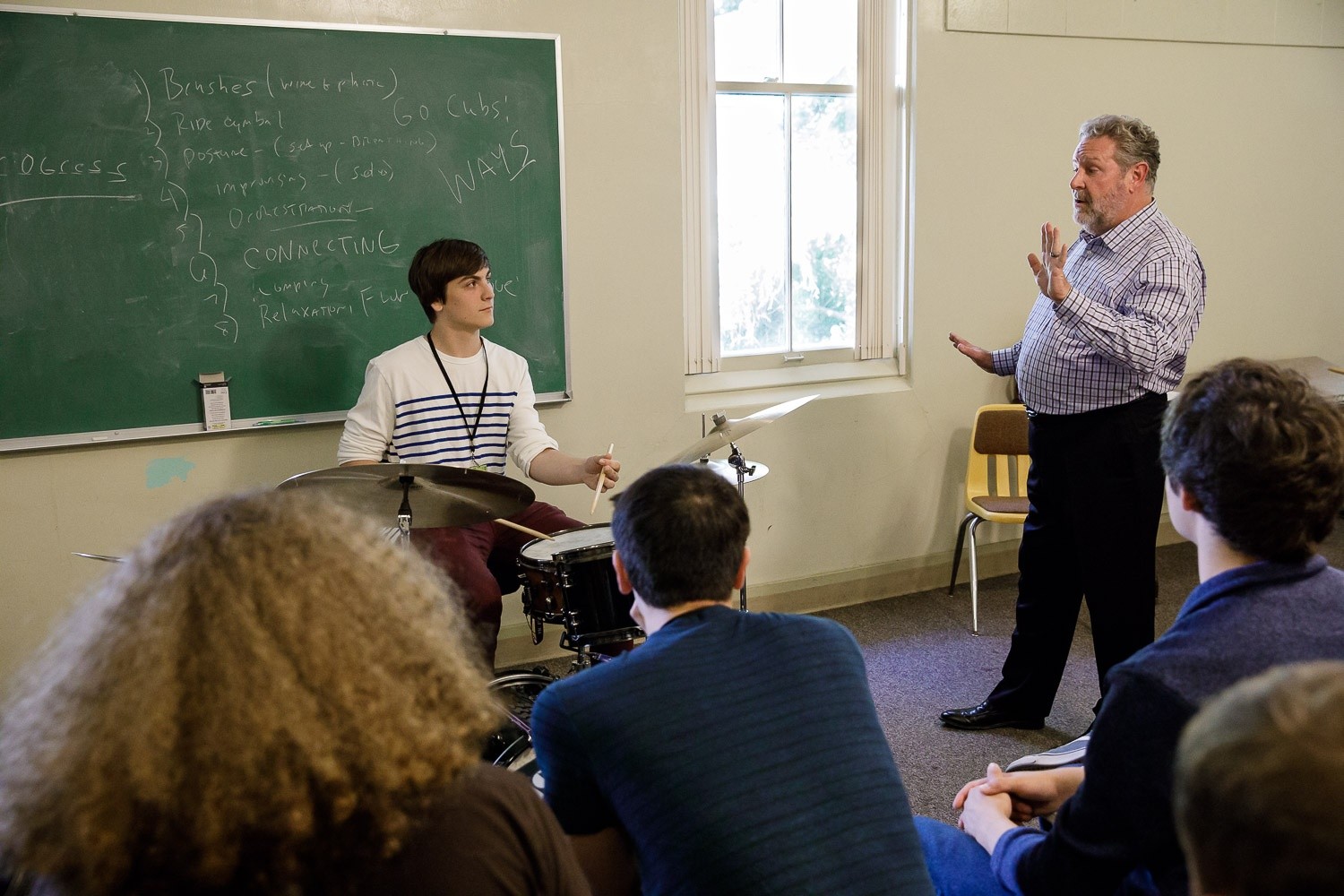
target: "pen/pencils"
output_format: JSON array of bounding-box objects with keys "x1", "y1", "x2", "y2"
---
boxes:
[{"x1": 251, "y1": 418, "x2": 306, "y2": 427}]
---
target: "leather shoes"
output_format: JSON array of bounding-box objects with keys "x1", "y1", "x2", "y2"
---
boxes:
[{"x1": 938, "y1": 699, "x2": 1045, "y2": 730}]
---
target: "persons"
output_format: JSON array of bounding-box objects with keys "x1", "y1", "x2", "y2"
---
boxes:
[
  {"x1": 913, "y1": 357, "x2": 1344, "y2": 896},
  {"x1": 339, "y1": 237, "x2": 620, "y2": 672},
  {"x1": 532, "y1": 462, "x2": 930, "y2": 895},
  {"x1": 1170, "y1": 657, "x2": 1344, "y2": 896},
  {"x1": 936, "y1": 114, "x2": 1205, "y2": 735},
  {"x1": 0, "y1": 487, "x2": 587, "y2": 895}
]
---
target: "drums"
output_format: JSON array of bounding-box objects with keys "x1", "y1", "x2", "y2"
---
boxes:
[
  {"x1": 518, "y1": 521, "x2": 616, "y2": 626},
  {"x1": 550, "y1": 540, "x2": 647, "y2": 648},
  {"x1": 473, "y1": 668, "x2": 649, "y2": 896}
]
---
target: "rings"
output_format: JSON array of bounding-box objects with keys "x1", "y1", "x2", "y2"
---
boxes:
[{"x1": 1050, "y1": 253, "x2": 1060, "y2": 257}]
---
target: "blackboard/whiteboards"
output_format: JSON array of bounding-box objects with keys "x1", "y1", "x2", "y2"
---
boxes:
[{"x1": 0, "y1": 5, "x2": 568, "y2": 452}]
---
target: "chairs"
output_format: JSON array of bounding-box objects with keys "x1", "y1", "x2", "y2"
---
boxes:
[{"x1": 946, "y1": 403, "x2": 1032, "y2": 639}]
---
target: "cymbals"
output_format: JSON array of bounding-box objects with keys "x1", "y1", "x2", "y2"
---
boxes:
[
  {"x1": 608, "y1": 392, "x2": 821, "y2": 503},
  {"x1": 272, "y1": 461, "x2": 536, "y2": 529},
  {"x1": 70, "y1": 551, "x2": 126, "y2": 565},
  {"x1": 687, "y1": 458, "x2": 769, "y2": 486}
]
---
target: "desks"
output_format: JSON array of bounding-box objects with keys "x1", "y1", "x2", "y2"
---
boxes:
[{"x1": 1163, "y1": 355, "x2": 1344, "y2": 409}]
